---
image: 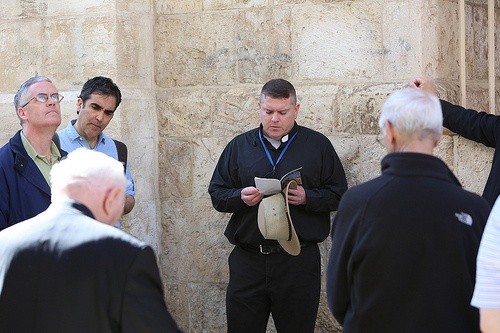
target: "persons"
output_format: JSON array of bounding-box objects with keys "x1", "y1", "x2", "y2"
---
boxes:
[
  {"x1": 470, "y1": 195, "x2": 500, "y2": 333},
  {"x1": 326, "y1": 88, "x2": 492, "y2": 333},
  {"x1": 410, "y1": 78, "x2": 500, "y2": 205},
  {"x1": 208, "y1": 79, "x2": 348, "y2": 333},
  {"x1": 0, "y1": 148, "x2": 183, "y2": 333},
  {"x1": 57, "y1": 76, "x2": 136, "y2": 228},
  {"x1": 0, "y1": 76, "x2": 69, "y2": 231}
]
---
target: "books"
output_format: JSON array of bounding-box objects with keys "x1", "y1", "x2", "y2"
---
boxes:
[{"x1": 255, "y1": 167, "x2": 304, "y2": 195}]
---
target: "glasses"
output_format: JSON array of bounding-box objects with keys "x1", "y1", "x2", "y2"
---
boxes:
[
  {"x1": 376, "y1": 127, "x2": 384, "y2": 147},
  {"x1": 22, "y1": 93, "x2": 64, "y2": 108}
]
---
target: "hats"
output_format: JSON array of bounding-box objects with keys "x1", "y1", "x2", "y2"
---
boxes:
[{"x1": 257, "y1": 179, "x2": 302, "y2": 256}]
